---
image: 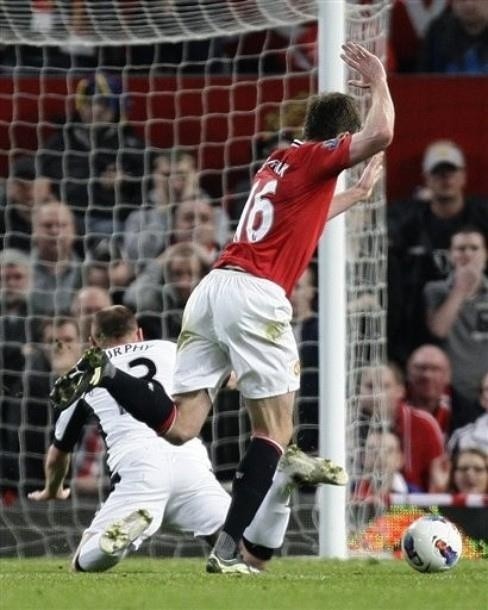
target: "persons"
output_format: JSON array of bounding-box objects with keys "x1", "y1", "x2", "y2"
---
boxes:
[
  {"x1": 399, "y1": 345, "x2": 487, "y2": 433},
  {"x1": 46, "y1": 40, "x2": 396, "y2": 574},
  {"x1": 353, "y1": 367, "x2": 488, "y2": 538},
  {"x1": 387, "y1": 140, "x2": 487, "y2": 364},
  {"x1": 23, "y1": 302, "x2": 350, "y2": 573},
  {"x1": 423, "y1": 224, "x2": 487, "y2": 428},
  {"x1": 2, "y1": 72, "x2": 321, "y2": 541},
  {"x1": 2, "y1": 2, "x2": 488, "y2": 74}
]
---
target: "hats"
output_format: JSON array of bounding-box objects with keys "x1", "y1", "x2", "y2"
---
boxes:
[
  {"x1": 76, "y1": 76, "x2": 121, "y2": 107},
  {"x1": 422, "y1": 140, "x2": 463, "y2": 173}
]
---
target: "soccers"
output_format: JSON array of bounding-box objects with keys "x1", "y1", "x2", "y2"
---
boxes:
[{"x1": 403, "y1": 517, "x2": 462, "y2": 573}]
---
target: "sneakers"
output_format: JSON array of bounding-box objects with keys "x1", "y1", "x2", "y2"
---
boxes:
[
  {"x1": 49, "y1": 347, "x2": 109, "y2": 411},
  {"x1": 280, "y1": 444, "x2": 350, "y2": 485},
  {"x1": 100, "y1": 509, "x2": 152, "y2": 552},
  {"x1": 207, "y1": 552, "x2": 258, "y2": 574}
]
